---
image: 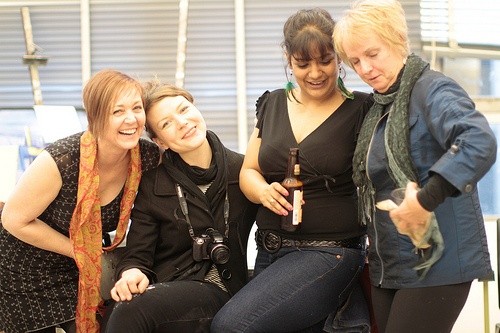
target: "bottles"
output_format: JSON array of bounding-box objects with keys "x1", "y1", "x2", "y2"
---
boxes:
[{"x1": 280, "y1": 147, "x2": 303, "y2": 232}]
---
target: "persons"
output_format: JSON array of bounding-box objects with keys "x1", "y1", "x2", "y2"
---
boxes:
[
  {"x1": 330, "y1": 0, "x2": 497, "y2": 333},
  {"x1": 213, "y1": 8, "x2": 376, "y2": 333},
  {"x1": 0, "y1": 69, "x2": 161, "y2": 333},
  {"x1": 111, "y1": 84, "x2": 252, "y2": 304}
]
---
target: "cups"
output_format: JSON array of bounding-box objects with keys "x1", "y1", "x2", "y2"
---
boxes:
[{"x1": 391, "y1": 187, "x2": 412, "y2": 207}]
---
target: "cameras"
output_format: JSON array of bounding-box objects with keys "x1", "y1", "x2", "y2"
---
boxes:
[{"x1": 192, "y1": 228, "x2": 231, "y2": 265}]
